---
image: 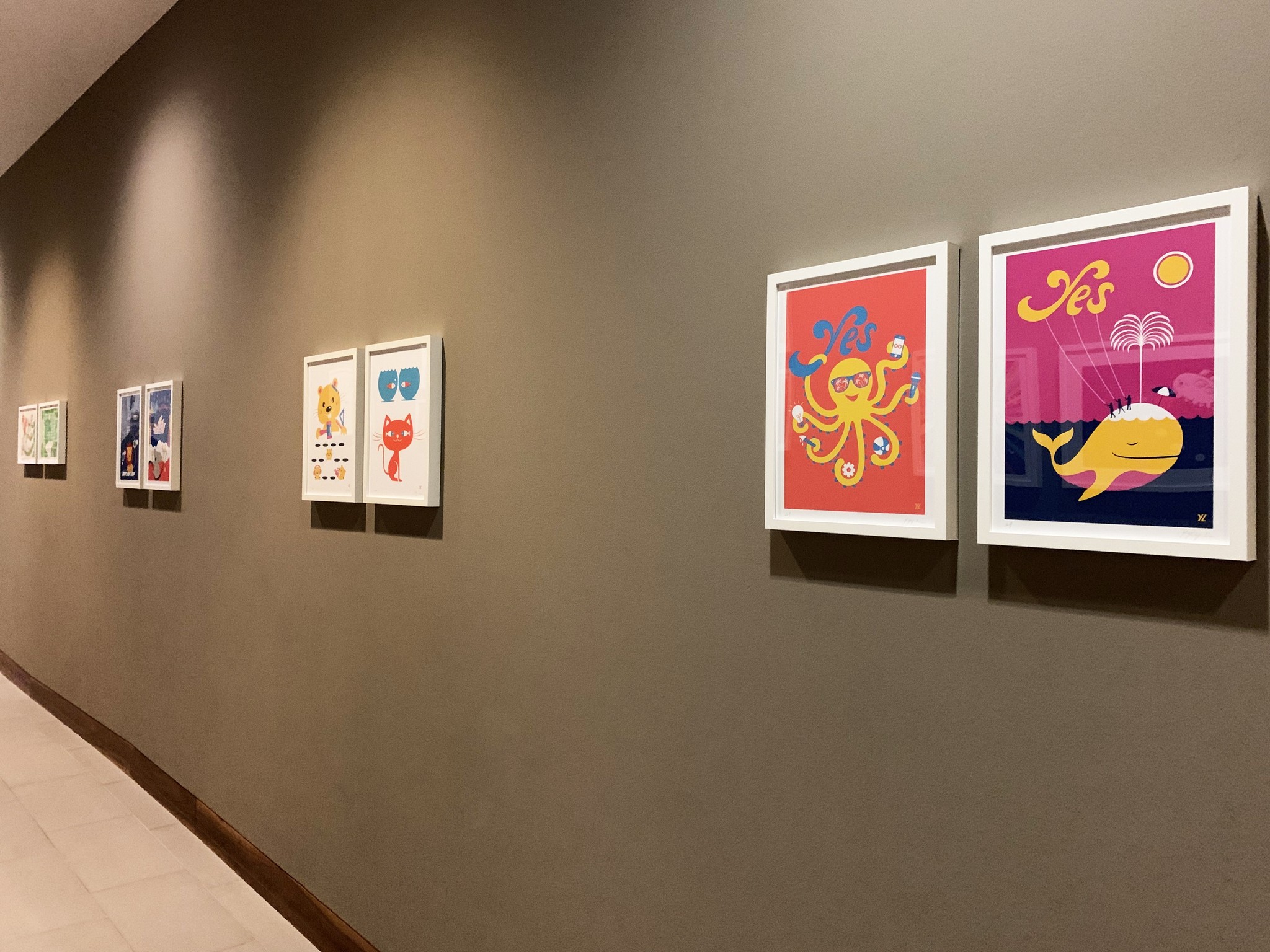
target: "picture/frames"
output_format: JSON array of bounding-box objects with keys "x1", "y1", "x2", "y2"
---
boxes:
[
  {"x1": 17, "y1": 404, "x2": 38, "y2": 464},
  {"x1": 142, "y1": 379, "x2": 182, "y2": 491},
  {"x1": 37, "y1": 400, "x2": 66, "y2": 466},
  {"x1": 301, "y1": 347, "x2": 363, "y2": 503},
  {"x1": 759, "y1": 240, "x2": 962, "y2": 548},
  {"x1": 115, "y1": 386, "x2": 144, "y2": 490},
  {"x1": 362, "y1": 334, "x2": 441, "y2": 508},
  {"x1": 976, "y1": 185, "x2": 1266, "y2": 565}
]
